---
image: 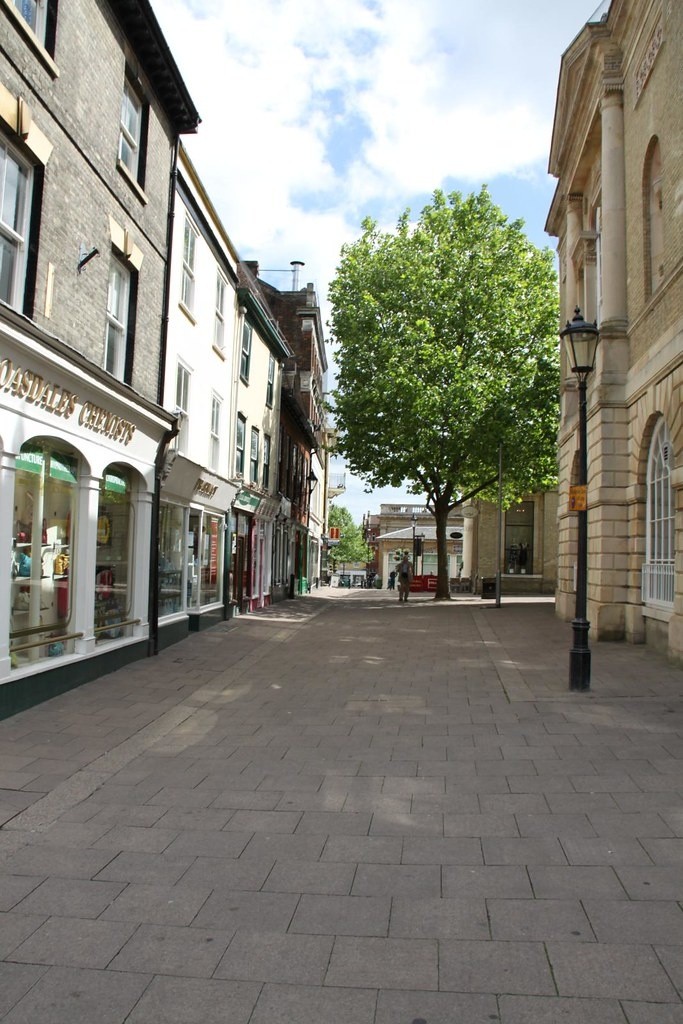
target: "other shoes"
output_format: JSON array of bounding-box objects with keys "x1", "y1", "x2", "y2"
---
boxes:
[
  {"x1": 399, "y1": 598, "x2": 403, "y2": 601},
  {"x1": 405, "y1": 599, "x2": 408, "y2": 602}
]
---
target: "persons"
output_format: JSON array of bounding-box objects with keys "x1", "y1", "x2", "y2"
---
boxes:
[
  {"x1": 396, "y1": 555, "x2": 414, "y2": 601},
  {"x1": 13, "y1": 548, "x2": 31, "y2": 576},
  {"x1": 508, "y1": 538, "x2": 519, "y2": 572},
  {"x1": 388, "y1": 573, "x2": 395, "y2": 590},
  {"x1": 519, "y1": 538, "x2": 530, "y2": 573},
  {"x1": 368, "y1": 573, "x2": 374, "y2": 588}
]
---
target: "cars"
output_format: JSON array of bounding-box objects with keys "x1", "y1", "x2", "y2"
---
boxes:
[{"x1": 329, "y1": 576, "x2": 349, "y2": 586}]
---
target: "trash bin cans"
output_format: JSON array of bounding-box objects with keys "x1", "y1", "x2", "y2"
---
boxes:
[
  {"x1": 481, "y1": 576, "x2": 497, "y2": 599},
  {"x1": 374, "y1": 577, "x2": 382, "y2": 588}
]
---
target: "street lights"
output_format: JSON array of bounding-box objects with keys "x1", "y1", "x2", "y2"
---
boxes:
[
  {"x1": 557, "y1": 308, "x2": 600, "y2": 689},
  {"x1": 366, "y1": 528, "x2": 371, "y2": 582},
  {"x1": 411, "y1": 514, "x2": 417, "y2": 576}
]
